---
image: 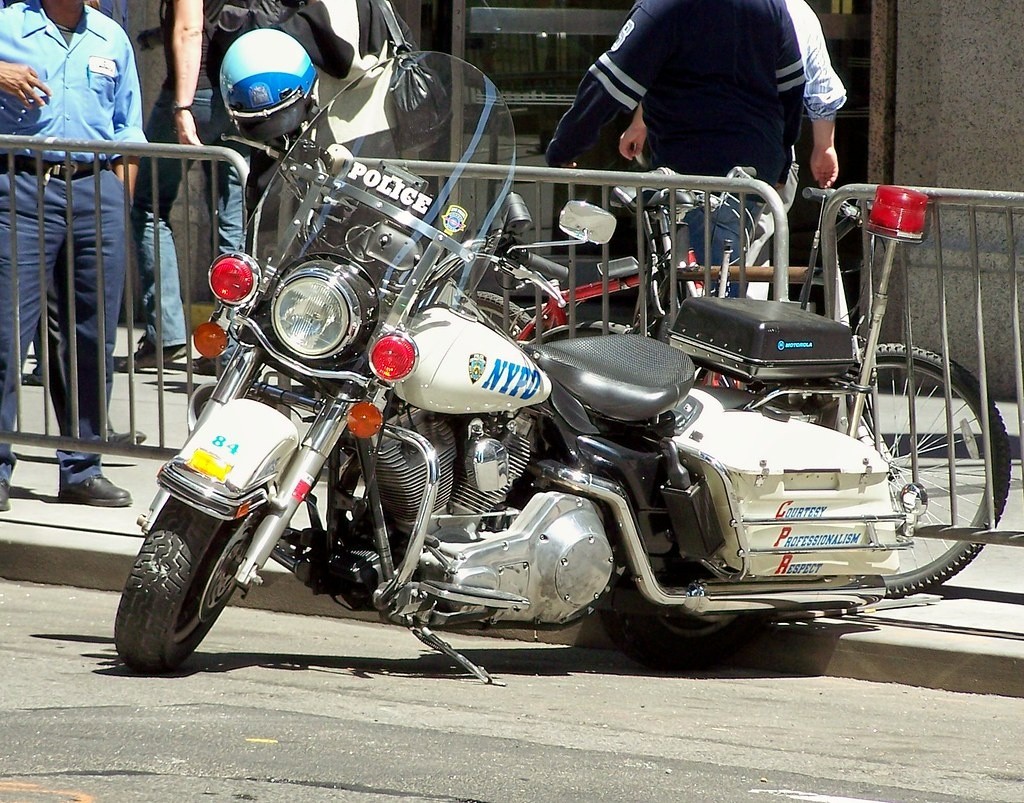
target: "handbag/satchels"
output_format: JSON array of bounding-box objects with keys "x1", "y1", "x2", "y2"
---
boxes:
[{"x1": 375, "y1": 0, "x2": 451, "y2": 146}]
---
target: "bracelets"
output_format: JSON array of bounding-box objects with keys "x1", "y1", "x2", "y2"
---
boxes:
[{"x1": 172, "y1": 102, "x2": 192, "y2": 114}]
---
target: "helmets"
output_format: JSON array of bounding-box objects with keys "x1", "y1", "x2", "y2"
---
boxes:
[{"x1": 220, "y1": 29, "x2": 317, "y2": 141}]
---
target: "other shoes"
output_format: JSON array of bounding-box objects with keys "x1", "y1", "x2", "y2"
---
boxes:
[
  {"x1": 108, "y1": 430, "x2": 146, "y2": 444},
  {"x1": 192, "y1": 357, "x2": 216, "y2": 375},
  {"x1": 120, "y1": 332, "x2": 187, "y2": 372},
  {"x1": 22, "y1": 374, "x2": 45, "y2": 385}
]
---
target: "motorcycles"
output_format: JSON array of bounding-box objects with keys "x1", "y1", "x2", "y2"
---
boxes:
[{"x1": 113, "y1": 47, "x2": 929, "y2": 685}]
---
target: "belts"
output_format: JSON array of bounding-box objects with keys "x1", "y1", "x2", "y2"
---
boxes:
[{"x1": 0, "y1": 153, "x2": 111, "y2": 180}]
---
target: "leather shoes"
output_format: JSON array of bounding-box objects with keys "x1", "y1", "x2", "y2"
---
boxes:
[
  {"x1": 59, "y1": 475, "x2": 131, "y2": 506},
  {"x1": 0, "y1": 478, "x2": 10, "y2": 511}
]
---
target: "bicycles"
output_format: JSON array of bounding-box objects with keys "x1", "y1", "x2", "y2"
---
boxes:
[{"x1": 465, "y1": 180, "x2": 1013, "y2": 596}]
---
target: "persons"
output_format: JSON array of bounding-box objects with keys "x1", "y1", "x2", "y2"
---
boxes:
[
  {"x1": 0, "y1": 0, "x2": 147, "y2": 512},
  {"x1": 117, "y1": 1, "x2": 412, "y2": 376},
  {"x1": 545, "y1": 0, "x2": 846, "y2": 389}
]
